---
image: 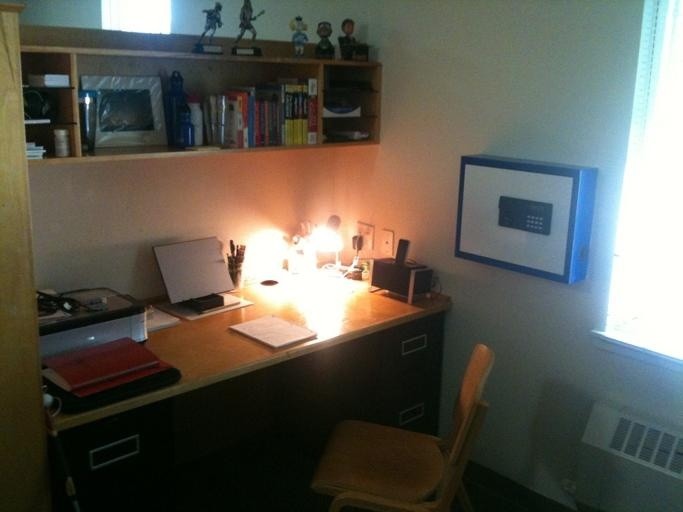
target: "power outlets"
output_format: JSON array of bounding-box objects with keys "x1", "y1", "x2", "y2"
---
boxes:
[{"x1": 356, "y1": 221, "x2": 375, "y2": 252}]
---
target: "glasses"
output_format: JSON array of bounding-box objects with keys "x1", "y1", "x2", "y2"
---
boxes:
[{"x1": 36, "y1": 290, "x2": 80, "y2": 312}]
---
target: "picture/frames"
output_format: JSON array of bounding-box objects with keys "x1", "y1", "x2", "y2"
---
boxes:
[{"x1": 80, "y1": 73, "x2": 171, "y2": 150}]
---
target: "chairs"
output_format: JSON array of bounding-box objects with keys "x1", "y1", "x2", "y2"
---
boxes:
[{"x1": 310, "y1": 342, "x2": 496, "y2": 512}]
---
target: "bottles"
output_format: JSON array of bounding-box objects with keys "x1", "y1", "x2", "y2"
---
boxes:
[
  {"x1": 162, "y1": 70, "x2": 195, "y2": 149},
  {"x1": 187, "y1": 95, "x2": 204, "y2": 147},
  {"x1": 54, "y1": 129, "x2": 70, "y2": 158}
]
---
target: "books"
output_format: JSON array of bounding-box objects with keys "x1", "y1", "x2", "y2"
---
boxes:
[
  {"x1": 227, "y1": 313, "x2": 316, "y2": 348},
  {"x1": 28, "y1": 73, "x2": 69, "y2": 88},
  {"x1": 227, "y1": 76, "x2": 320, "y2": 146},
  {"x1": 36, "y1": 336, "x2": 179, "y2": 416},
  {"x1": 26, "y1": 140, "x2": 46, "y2": 160}
]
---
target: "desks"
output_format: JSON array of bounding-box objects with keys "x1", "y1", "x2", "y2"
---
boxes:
[{"x1": 39, "y1": 267, "x2": 454, "y2": 510}]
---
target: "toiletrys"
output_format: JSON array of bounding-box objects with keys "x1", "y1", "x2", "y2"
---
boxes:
[{"x1": 79, "y1": 89, "x2": 97, "y2": 156}]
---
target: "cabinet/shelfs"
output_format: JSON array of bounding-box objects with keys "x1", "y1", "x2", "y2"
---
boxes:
[{"x1": 18, "y1": 24, "x2": 383, "y2": 167}]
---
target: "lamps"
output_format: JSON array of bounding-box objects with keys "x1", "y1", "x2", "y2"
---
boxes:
[
  {"x1": 246, "y1": 225, "x2": 299, "y2": 286},
  {"x1": 309, "y1": 215, "x2": 345, "y2": 279}
]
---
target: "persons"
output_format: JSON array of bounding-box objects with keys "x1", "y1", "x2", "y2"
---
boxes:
[
  {"x1": 235, "y1": 0, "x2": 265, "y2": 42},
  {"x1": 197, "y1": 1, "x2": 226, "y2": 49},
  {"x1": 289, "y1": 16, "x2": 309, "y2": 59},
  {"x1": 336, "y1": 17, "x2": 366, "y2": 61},
  {"x1": 314, "y1": 21, "x2": 335, "y2": 60}
]
